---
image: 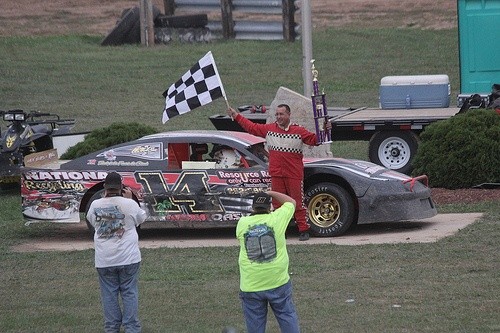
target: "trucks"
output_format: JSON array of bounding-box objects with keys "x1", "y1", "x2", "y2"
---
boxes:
[{"x1": 208, "y1": 0, "x2": 500, "y2": 175}]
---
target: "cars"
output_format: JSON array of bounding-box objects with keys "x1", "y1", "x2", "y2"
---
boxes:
[{"x1": 20, "y1": 129, "x2": 437, "y2": 236}]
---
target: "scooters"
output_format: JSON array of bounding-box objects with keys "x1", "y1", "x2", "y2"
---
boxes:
[{"x1": 1, "y1": 110, "x2": 79, "y2": 187}]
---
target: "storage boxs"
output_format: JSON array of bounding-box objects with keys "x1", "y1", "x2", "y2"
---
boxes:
[{"x1": 379, "y1": 74, "x2": 451, "y2": 109}]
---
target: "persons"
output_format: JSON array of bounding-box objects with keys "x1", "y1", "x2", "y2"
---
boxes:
[
  {"x1": 235, "y1": 189, "x2": 301, "y2": 333},
  {"x1": 85, "y1": 171, "x2": 148, "y2": 332},
  {"x1": 226, "y1": 104, "x2": 332, "y2": 241}
]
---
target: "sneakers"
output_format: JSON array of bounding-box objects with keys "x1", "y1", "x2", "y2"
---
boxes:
[{"x1": 299, "y1": 232, "x2": 311, "y2": 240}]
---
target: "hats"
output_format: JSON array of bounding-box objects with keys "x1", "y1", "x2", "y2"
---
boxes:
[
  {"x1": 104, "y1": 171, "x2": 121, "y2": 189},
  {"x1": 252, "y1": 193, "x2": 271, "y2": 209}
]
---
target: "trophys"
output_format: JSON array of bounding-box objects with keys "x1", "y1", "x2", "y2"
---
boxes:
[{"x1": 305, "y1": 59, "x2": 334, "y2": 145}]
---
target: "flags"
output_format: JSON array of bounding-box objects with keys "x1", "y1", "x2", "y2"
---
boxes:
[{"x1": 161, "y1": 51, "x2": 224, "y2": 124}]
